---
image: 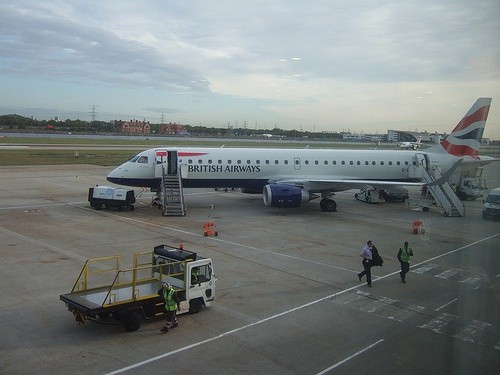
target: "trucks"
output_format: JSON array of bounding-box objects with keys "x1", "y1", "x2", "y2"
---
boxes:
[{"x1": 450, "y1": 176, "x2": 483, "y2": 202}]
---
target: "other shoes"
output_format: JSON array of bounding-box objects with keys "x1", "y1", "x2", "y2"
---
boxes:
[
  {"x1": 402, "y1": 280, "x2": 406, "y2": 283},
  {"x1": 160, "y1": 328, "x2": 168, "y2": 332},
  {"x1": 400, "y1": 272, "x2": 402, "y2": 278},
  {"x1": 368, "y1": 284, "x2": 372, "y2": 287},
  {"x1": 171, "y1": 324, "x2": 178, "y2": 328},
  {"x1": 358, "y1": 274, "x2": 362, "y2": 281}
]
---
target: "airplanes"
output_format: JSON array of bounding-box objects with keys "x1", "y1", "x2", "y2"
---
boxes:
[
  {"x1": 396, "y1": 136, "x2": 424, "y2": 150},
  {"x1": 106, "y1": 97, "x2": 500, "y2": 216}
]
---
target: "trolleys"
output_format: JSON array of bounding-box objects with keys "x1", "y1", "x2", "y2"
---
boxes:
[
  {"x1": 203, "y1": 219, "x2": 219, "y2": 237},
  {"x1": 412, "y1": 216, "x2": 426, "y2": 235}
]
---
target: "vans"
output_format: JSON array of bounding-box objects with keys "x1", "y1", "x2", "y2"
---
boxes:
[{"x1": 482, "y1": 187, "x2": 500, "y2": 220}]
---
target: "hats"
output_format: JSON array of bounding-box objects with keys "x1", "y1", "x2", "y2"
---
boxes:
[{"x1": 163, "y1": 282, "x2": 171, "y2": 288}]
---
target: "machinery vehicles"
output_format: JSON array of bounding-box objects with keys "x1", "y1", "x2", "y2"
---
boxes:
[
  {"x1": 87, "y1": 185, "x2": 136, "y2": 212},
  {"x1": 59, "y1": 244, "x2": 217, "y2": 332}
]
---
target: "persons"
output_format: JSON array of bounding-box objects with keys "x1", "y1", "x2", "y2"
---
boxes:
[
  {"x1": 157, "y1": 282, "x2": 180, "y2": 333},
  {"x1": 191, "y1": 267, "x2": 199, "y2": 285},
  {"x1": 397, "y1": 242, "x2": 413, "y2": 283},
  {"x1": 451, "y1": 183, "x2": 456, "y2": 193},
  {"x1": 358, "y1": 240, "x2": 374, "y2": 288}
]
